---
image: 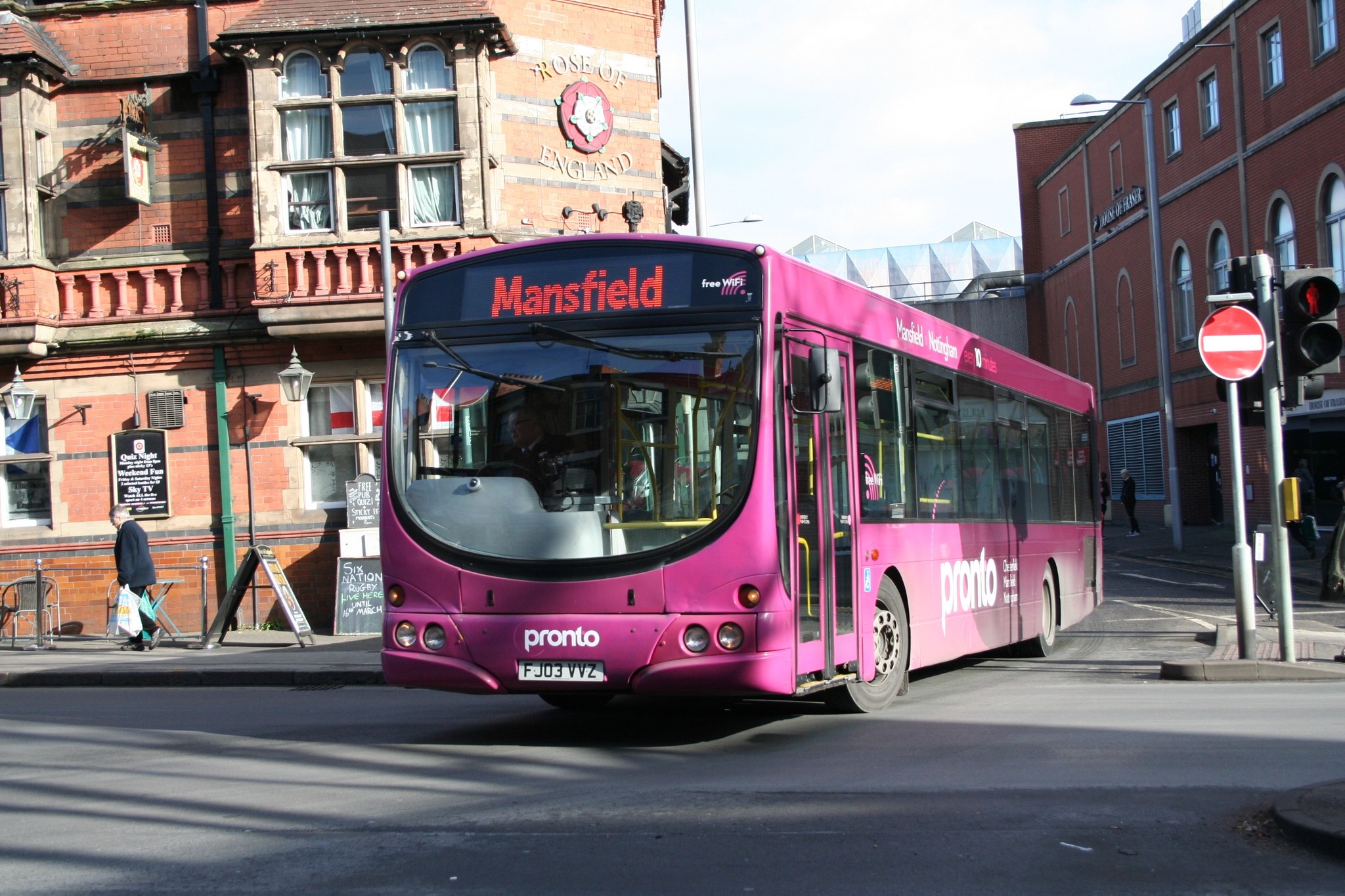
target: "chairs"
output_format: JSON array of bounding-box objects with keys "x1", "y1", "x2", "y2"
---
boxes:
[
  {"x1": 0, "y1": 576, "x2": 61, "y2": 648},
  {"x1": 106, "y1": 579, "x2": 154, "y2": 642}
]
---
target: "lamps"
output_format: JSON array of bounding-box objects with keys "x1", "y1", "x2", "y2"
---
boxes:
[
  {"x1": 276, "y1": 336, "x2": 316, "y2": 401},
  {"x1": 0, "y1": 357, "x2": 38, "y2": 420},
  {"x1": 563, "y1": 203, "x2": 600, "y2": 218}
]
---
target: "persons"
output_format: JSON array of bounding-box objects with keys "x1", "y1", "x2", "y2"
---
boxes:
[
  {"x1": 110, "y1": 504, "x2": 165, "y2": 651},
  {"x1": 1319, "y1": 479, "x2": 1345, "y2": 663},
  {"x1": 1286, "y1": 458, "x2": 1315, "y2": 557},
  {"x1": 501, "y1": 405, "x2": 574, "y2": 464},
  {"x1": 1120, "y1": 468, "x2": 1138, "y2": 536},
  {"x1": 1099, "y1": 472, "x2": 1110, "y2": 539}
]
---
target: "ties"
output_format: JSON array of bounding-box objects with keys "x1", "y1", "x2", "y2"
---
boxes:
[{"x1": 523, "y1": 448, "x2": 531, "y2": 459}]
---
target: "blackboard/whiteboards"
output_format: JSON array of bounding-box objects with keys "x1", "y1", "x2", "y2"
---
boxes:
[
  {"x1": 345, "y1": 472, "x2": 380, "y2": 529},
  {"x1": 333, "y1": 556, "x2": 384, "y2": 635}
]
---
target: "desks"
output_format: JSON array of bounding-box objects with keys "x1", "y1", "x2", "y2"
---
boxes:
[{"x1": 146, "y1": 579, "x2": 184, "y2": 642}]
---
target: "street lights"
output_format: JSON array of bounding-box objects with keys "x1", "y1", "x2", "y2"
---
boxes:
[{"x1": 1070, "y1": 92, "x2": 1185, "y2": 553}]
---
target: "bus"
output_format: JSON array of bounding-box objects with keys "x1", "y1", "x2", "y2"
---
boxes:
[{"x1": 381, "y1": 226, "x2": 1106, "y2": 713}]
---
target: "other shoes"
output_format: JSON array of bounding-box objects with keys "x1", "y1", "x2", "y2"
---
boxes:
[
  {"x1": 1126, "y1": 531, "x2": 1140, "y2": 536},
  {"x1": 1102, "y1": 535, "x2": 1106, "y2": 539}
]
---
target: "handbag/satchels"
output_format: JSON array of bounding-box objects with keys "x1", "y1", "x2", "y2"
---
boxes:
[{"x1": 108, "y1": 584, "x2": 143, "y2": 638}]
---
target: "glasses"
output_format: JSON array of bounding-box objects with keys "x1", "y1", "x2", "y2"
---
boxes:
[{"x1": 507, "y1": 418, "x2": 532, "y2": 433}]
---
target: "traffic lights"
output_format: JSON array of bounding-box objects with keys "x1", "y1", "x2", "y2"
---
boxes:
[
  {"x1": 1281, "y1": 375, "x2": 1325, "y2": 403},
  {"x1": 1283, "y1": 266, "x2": 1342, "y2": 379}
]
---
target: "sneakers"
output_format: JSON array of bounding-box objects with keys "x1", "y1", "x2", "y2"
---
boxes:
[
  {"x1": 149, "y1": 627, "x2": 165, "y2": 650},
  {"x1": 120, "y1": 640, "x2": 145, "y2": 651}
]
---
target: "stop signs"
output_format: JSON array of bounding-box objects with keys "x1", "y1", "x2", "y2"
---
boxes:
[{"x1": 1198, "y1": 304, "x2": 1269, "y2": 384}]
